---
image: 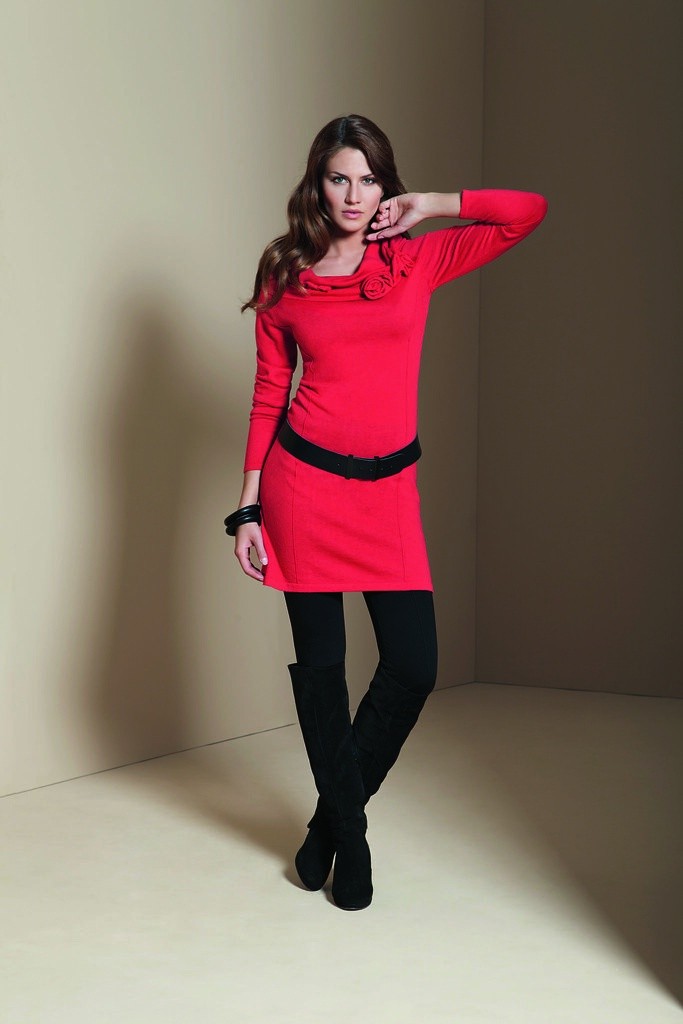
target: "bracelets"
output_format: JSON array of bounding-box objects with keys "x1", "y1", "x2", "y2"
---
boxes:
[
  {"x1": 223, "y1": 504, "x2": 261, "y2": 525},
  {"x1": 225, "y1": 512, "x2": 260, "y2": 536}
]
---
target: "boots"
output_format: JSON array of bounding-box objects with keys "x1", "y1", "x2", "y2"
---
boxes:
[
  {"x1": 295, "y1": 662, "x2": 433, "y2": 890},
  {"x1": 288, "y1": 662, "x2": 373, "y2": 911}
]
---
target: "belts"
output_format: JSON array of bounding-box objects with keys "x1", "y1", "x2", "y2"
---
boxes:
[{"x1": 276, "y1": 418, "x2": 423, "y2": 481}]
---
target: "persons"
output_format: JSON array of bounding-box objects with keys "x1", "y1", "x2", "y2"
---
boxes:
[{"x1": 224, "y1": 114, "x2": 547, "y2": 912}]
